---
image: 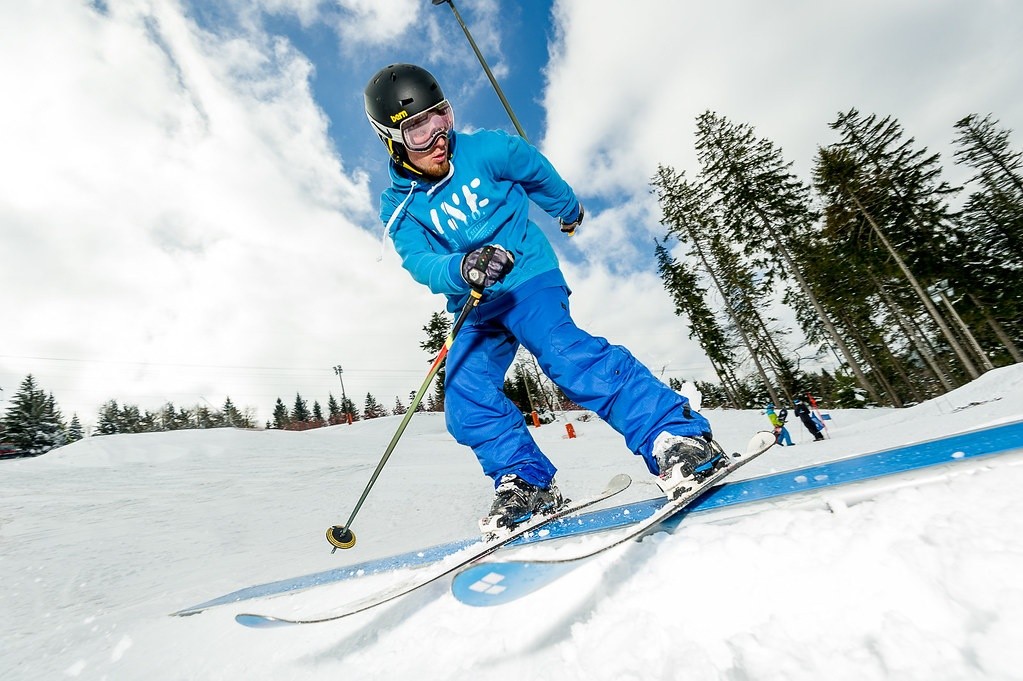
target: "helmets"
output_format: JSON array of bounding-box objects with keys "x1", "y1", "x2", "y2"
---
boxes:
[
  {"x1": 365, "y1": 63, "x2": 445, "y2": 165},
  {"x1": 767, "y1": 403, "x2": 774, "y2": 412},
  {"x1": 794, "y1": 399, "x2": 802, "y2": 405}
]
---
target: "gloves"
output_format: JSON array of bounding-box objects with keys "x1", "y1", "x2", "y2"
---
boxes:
[
  {"x1": 462, "y1": 244, "x2": 515, "y2": 286},
  {"x1": 557, "y1": 200, "x2": 583, "y2": 233}
]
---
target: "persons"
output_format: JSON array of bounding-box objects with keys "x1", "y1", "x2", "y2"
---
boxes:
[
  {"x1": 794, "y1": 399, "x2": 824, "y2": 442},
  {"x1": 364, "y1": 64, "x2": 730, "y2": 540},
  {"x1": 766, "y1": 404, "x2": 796, "y2": 446}
]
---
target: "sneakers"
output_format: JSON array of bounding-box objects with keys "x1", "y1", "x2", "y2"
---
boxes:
[
  {"x1": 477, "y1": 473, "x2": 562, "y2": 533},
  {"x1": 650, "y1": 430, "x2": 730, "y2": 500}
]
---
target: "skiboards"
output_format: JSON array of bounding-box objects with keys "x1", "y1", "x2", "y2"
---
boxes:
[{"x1": 235, "y1": 430, "x2": 777, "y2": 628}]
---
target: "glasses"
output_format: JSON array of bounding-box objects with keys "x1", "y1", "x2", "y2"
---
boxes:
[{"x1": 399, "y1": 99, "x2": 455, "y2": 154}]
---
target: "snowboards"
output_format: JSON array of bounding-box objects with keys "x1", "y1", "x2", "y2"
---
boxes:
[{"x1": 771, "y1": 408, "x2": 788, "y2": 443}]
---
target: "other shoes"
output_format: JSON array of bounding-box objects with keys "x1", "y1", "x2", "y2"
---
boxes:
[
  {"x1": 777, "y1": 443, "x2": 784, "y2": 446},
  {"x1": 814, "y1": 438, "x2": 824, "y2": 441},
  {"x1": 788, "y1": 443, "x2": 795, "y2": 446}
]
